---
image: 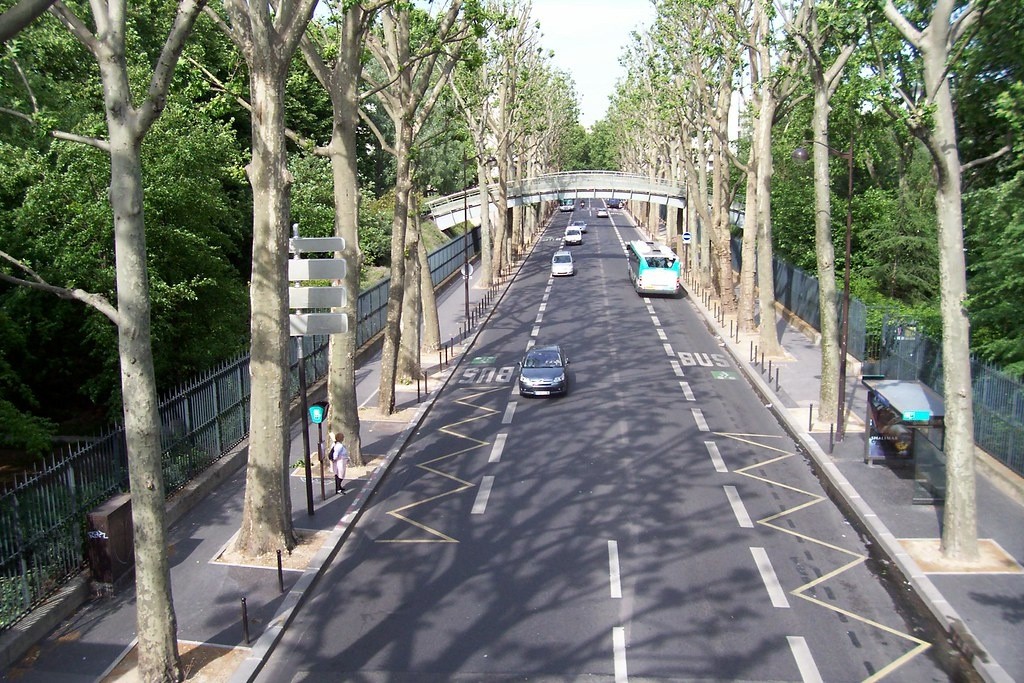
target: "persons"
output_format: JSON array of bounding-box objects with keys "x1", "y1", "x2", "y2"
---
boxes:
[
  {"x1": 328, "y1": 432, "x2": 349, "y2": 496},
  {"x1": 426, "y1": 181, "x2": 434, "y2": 196},
  {"x1": 580, "y1": 199, "x2": 585, "y2": 204}
]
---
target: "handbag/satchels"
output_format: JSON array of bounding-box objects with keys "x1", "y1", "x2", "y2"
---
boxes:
[{"x1": 329, "y1": 447, "x2": 334, "y2": 461}]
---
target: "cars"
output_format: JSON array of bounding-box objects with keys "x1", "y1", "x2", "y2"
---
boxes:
[
  {"x1": 517, "y1": 345, "x2": 571, "y2": 398},
  {"x1": 607, "y1": 198, "x2": 621, "y2": 209},
  {"x1": 596, "y1": 208, "x2": 608, "y2": 217},
  {"x1": 571, "y1": 220, "x2": 587, "y2": 232},
  {"x1": 550, "y1": 250, "x2": 575, "y2": 276}
]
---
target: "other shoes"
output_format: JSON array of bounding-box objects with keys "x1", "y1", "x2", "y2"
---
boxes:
[
  {"x1": 336, "y1": 490, "x2": 346, "y2": 495},
  {"x1": 341, "y1": 488, "x2": 348, "y2": 491}
]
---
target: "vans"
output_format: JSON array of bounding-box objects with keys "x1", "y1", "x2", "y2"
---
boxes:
[{"x1": 562, "y1": 225, "x2": 582, "y2": 245}]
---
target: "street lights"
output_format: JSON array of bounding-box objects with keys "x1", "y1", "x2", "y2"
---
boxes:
[
  {"x1": 308, "y1": 401, "x2": 330, "y2": 501},
  {"x1": 791, "y1": 100, "x2": 853, "y2": 440},
  {"x1": 461, "y1": 147, "x2": 497, "y2": 325}
]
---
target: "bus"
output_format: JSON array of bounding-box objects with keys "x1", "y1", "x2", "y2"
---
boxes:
[
  {"x1": 623, "y1": 240, "x2": 680, "y2": 297},
  {"x1": 559, "y1": 198, "x2": 576, "y2": 212}
]
